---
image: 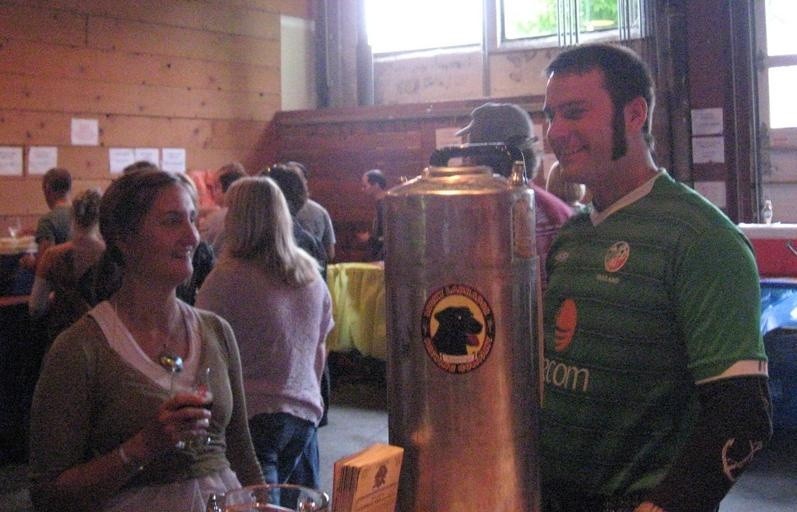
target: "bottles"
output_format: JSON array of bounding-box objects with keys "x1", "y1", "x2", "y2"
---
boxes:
[{"x1": 761, "y1": 199, "x2": 772, "y2": 224}]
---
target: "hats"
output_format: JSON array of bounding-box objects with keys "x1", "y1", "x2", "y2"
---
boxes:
[{"x1": 455, "y1": 101, "x2": 539, "y2": 152}]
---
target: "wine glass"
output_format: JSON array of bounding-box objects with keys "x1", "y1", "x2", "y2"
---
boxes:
[
  {"x1": 222, "y1": 482, "x2": 331, "y2": 511},
  {"x1": 8, "y1": 218, "x2": 22, "y2": 241},
  {"x1": 171, "y1": 365, "x2": 213, "y2": 457}
]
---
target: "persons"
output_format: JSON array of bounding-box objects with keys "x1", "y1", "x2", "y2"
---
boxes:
[
  {"x1": 458, "y1": 104, "x2": 587, "y2": 293},
  {"x1": 540, "y1": 44, "x2": 774, "y2": 511},
  {"x1": 354, "y1": 170, "x2": 387, "y2": 262},
  {"x1": 18, "y1": 159, "x2": 338, "y2": 512}
]
